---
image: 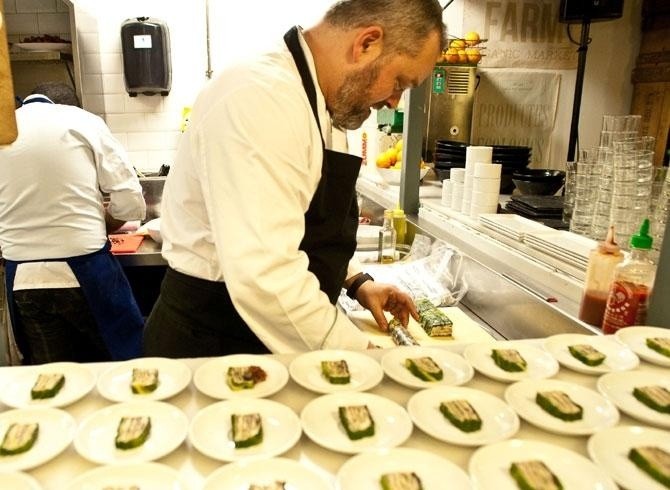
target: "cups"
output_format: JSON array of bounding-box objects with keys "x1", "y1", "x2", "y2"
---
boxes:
[{"x1": 565, "y1": 113, "x2": 670, "y2": 251}]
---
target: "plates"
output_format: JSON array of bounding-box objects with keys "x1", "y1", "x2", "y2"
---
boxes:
[
  {"x1": 469, "y1": 438, "x2": 621, "y2": 490},
  {"x1": 74, "y1": 401, "x2": 190, "y2": 461},
  {"x1": 300, "y1": 392, "x2": 414, "y2": 454},
  {"x1": 1, "y1": 362, "x2": 99, "y2": 407},
  {"x1": 190, "y1": 399, "x2": 302, "y2": 462},
  {"x1": 57, "y1": 461, "x2": 193, "y2": 490},
  {"x1": 0, "y1": 468, "x2": 42, "y2": 489},
  {"x1": 15, "y1": 42, "x2": 69, "y2": 53},
  {"x1": 194, "y1": 355, "x2": 289, "y2": 399},
  {"x1": 97, "y1": 357, "x2": 192, "y2": 402},
  {"x1": 335, "y1": 447, "x2": 477, "y2": 490},
  {"x1": 0, "y1": 407, "x2": 76, "y2": 472},
  {"x1": 288, "y1": 350, "x2": 384, "y2": 393},
  {"x1": 598, "y1": 367, "x2": 670, "y2": 431},
  {"x1": 381, "y1": 346, "x2": 475, "y2": 389},
  {"x1": 505, "y1": 191, "x2": 565, "y2": 219},
  {"x1": 546, "y1": 333, "x2": 640, "y2": 373},
  {"x1": 479, "y1": 214, "x2": 632, "y2": 276},
  {"x1": 613, "y1": 325, "x2": 670, "y2": 368},
  {"x1": 504, "y1": 380, "x2": 621, "y2": 436},
  {"x1": 587, "y1": 425, "x2": 670, "y2": 490},
  {"x1": 198, "y1": 454, "x2": 332, "y2": 490},
  {"x1": 408, "y1": 386, "x2": 519, "y2": 446},
  {"x1": 463, "y1": 342, "x2": 560, "y2": 384}
]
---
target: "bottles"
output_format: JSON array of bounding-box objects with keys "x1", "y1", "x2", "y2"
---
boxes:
[
  {"x1": 579, "y1": 227, "x2": 626, "y2": 326},
  {"x1": 386, "y1": 202, "x2": 406, "y2": 249},
  {"x1": 378, "y1": 209, "x2": 397, "y2": 264},
  {"x1": 602, "y1": 218, "x2": 658, "y2": 334}
]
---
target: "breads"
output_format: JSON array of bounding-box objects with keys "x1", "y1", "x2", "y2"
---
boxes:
[
  {"x1": 31, "y1": 373, "x2": 68, "y2": 399},
  {"x1": 322, "y1": 360, "x2": 351, "y2": 383},
  {"x1": 569, "y1": 344, "x2": 606, "y2": 365},
  {"x1": 1, "y1": 423, "x2": 39, "y2": 455},
  {"x1": 536, "y1": 390, "x2": 583, "y2": 421},
  {"x1": 128, "y1": 367, "x2": 162, "y2": 394},
  {"x1": 628, "y1": 446, "x2": 670, "y2": 486},
  {"x1": 492, "y1": 349, "x2": 527, "y2": 372},
  {"x1": 406, "y1": 356, "x2": 443, "y2": 381},
  {"x1": 380, "y1": 472, "x2": 426, "y2": 490},
  {"x1": 227, "y1": 367, "x2": 259, "y2": 390},
  {"x1": 231, "y1": 413, "x2": 264, "y2": 449},
  {"x1": 510, "y1": 460, "x2": 565, "y2": 490},
  {"x1": 338, "y1": 404, "x2": 376, "y2": 440},
  {"x1": 646, "y1": 337, "x2": 670, "y2": 356},
  {"x1": 114, "y1": 417, "x2": 153, "y2": 450},
  {"x1": 439, "y1": 399, "x2": 482, "y2": 432},
  {"x1": 632, "y1": 385, "x2": 670, "y2": 413}
]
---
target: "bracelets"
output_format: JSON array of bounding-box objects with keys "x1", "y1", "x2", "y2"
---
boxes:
[{"x1": 346, "y1": 274, "x2": 373, "y2": 299}]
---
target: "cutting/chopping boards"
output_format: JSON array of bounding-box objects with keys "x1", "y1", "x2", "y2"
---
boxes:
[
  {"x1": 348, "y1": 308, "x2": 500, "y2": 348},
  {"x1": 107, "y1": 235, "x2": 145, "y2": 254}
]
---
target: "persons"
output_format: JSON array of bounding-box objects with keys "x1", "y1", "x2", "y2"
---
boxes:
[
  {"x1": 1, "y1": 80, "x2": 146, "y2": 368},
  {"x1": 140, "y1": 0, "x2": 450, "y2": 356}
]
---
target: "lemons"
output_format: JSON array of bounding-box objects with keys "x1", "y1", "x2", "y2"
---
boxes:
[
  {"x1": 376, "y1": 137, "x2": 404, "y2": 171},
  {"x1": 437, "y1": 31, "x2": 481, "y2": 62}
]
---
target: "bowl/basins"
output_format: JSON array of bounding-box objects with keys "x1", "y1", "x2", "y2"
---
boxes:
[
  {"x1": 146, "y1": 226, "x2": 162, "y2": 243},
  {"x1": 355, "y1": 216, "x2": 386, "y2": 249},
  {"x1": 379, "y1": 166, "x2": 429, "y2": 187},
  {"x1": 440, "y1": 144, "x2": 501, "y2": 223},
  {"x1": 432, "y1": 138, "x2": 566, "y2": 196}
]
